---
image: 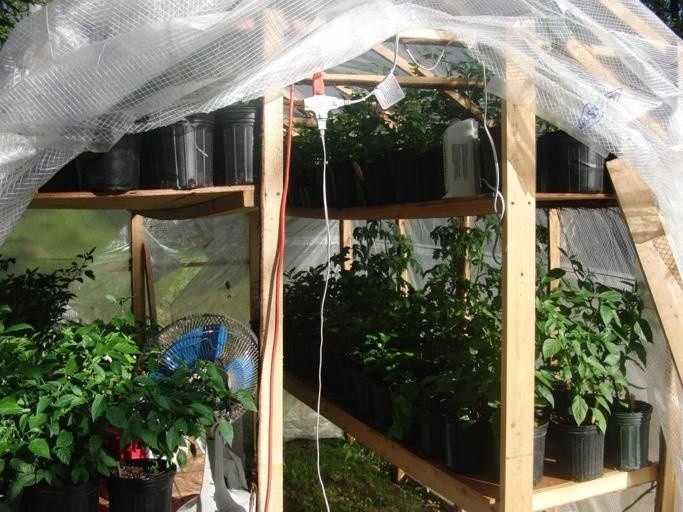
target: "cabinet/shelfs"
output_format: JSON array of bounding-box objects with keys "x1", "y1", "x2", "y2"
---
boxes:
[{"x1": 0, "y1": 0, "x2": 683, "y2": 512}]
